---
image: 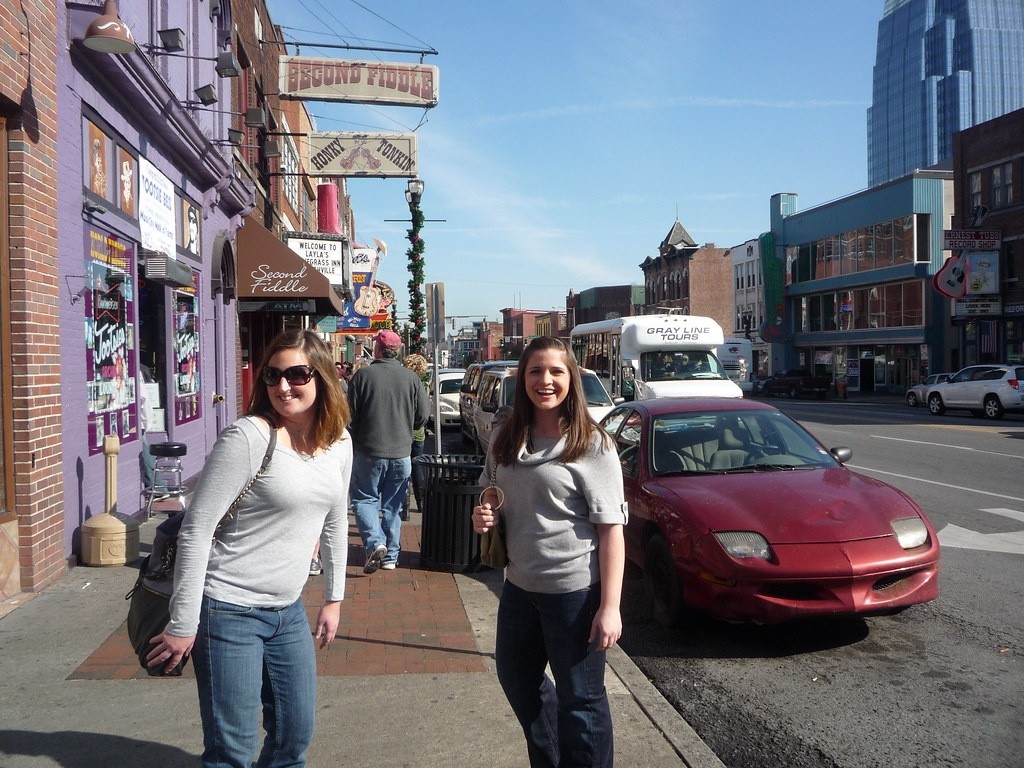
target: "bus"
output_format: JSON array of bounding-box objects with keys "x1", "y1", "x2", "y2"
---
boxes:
[
  {"x1": 704, "y1": 337, "x2": 753, "y2": 391},
  {"x1": 570, "y1": 315, "x2": 742, "y2": 403}
]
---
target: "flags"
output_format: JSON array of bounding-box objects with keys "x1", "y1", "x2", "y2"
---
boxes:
[{"x1": 979, "y1": 319, "x2": 997, "y2": 354}]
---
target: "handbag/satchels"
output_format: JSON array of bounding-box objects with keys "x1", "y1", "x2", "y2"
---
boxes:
[
  {"x1": 125, "y1": 512, "x2": 218, "y2": 676},
  {"x1": 480, "y1": 453, "x2": 508, "y2": 569}
]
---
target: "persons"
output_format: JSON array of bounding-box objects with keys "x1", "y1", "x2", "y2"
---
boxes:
[
  {"x1": 333, "y1": 358, "x2": 374, "y2": 399},
  {"x1": 347, "y1": 330, "x2": 430, "y2": 574},
  {"x1": 471, "y1": 335, "x2": 629, "y2": 768},
  {"x1": 309, "y1": 538, "x2": 323, "y2": 575},
  {"x1": 147, "y1": 330, "x2": 353, "y2": 768},
  {"x1": 400, "y1": 354, "x2": 434, "y2": 521}
]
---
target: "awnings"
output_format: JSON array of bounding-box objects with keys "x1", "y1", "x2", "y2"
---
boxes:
[{"x1": 235, "y1": 214, "x2": 344, "y2": 317}]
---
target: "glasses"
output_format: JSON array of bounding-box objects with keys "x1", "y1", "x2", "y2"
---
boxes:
[{"x1": 261, "y1": 365, "x2": 318, "y2": 386}]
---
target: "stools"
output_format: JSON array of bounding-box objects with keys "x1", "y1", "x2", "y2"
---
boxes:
[{"x1": 143, "y1": 441, "x2": 189, "y2": 519}]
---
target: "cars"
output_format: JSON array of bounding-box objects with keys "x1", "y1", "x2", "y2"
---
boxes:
[
  {"x1": 905, "y1": 373, "x2": 956, "y2": 408},
  {"x1": 598, "y1": 396, "x2": 940, "y2": 626},
  {"x1": 423, "y1": 363, "x2": 466, "y2": 432}
]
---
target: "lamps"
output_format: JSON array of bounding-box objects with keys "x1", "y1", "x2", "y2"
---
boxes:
[
  {"x1": 83, "y1": 1, "x2": 137, "y2": 54},
  {"x1": 177, "y1": 83, "x2": 219, "y2": 106},
  {"x1": 178, "y1": 107, "x2": 266, "y2": 129},
  {"x1": 220, "y1": 141, "x2": 282, "y2": 159},
  {"x1": 147, "y1": 52, "x2": 241, "y2": 77},
  {"x1": 209, "y1": 127, "x2": 245, "y2": 145},
  {"x1": 148, "y1": 28, "x2": 187, "y2": 53}
]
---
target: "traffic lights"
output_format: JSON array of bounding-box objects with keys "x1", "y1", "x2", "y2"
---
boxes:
[
  {"x1": 500, "y1": 339, "x2": 503, "y2": 347},
  {"x1": 525, "y1": 339, "x2": 528, "y2": 347}
]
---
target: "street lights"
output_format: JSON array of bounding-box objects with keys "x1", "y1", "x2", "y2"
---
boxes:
[{"x1": 404, "y1": 177, "x2": 425, "y2": 354}]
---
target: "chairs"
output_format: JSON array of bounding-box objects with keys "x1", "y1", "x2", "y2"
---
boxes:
[
  {"x1": 655, "y1": 430, "x2": 687, "y2": 471},
  {"x1": 709, "y1": 427, "x2": 755, "y2": 471}
]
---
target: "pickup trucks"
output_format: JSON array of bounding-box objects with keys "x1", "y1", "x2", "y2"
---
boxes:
[{"x1": 762, "y1": 368, "x2": 830, "y2": 400}]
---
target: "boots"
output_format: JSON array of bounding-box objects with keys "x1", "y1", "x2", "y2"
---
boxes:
[
  {"x1": 399, "y1": 481, "x2": 410, "y2": 521},
  {"x1": 414, "y1": 484, "x2": 423, "y2": 512}
]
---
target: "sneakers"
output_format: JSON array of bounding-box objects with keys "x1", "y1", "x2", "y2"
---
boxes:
[
  {"x1": 382, "y1": 562, "x2": 395, "y2": 570},
  {"x1": 309, "y1": 555, "x2": 321, "y2": 576},
  {"x1": 145, "y1": 493, "x2": 170, "y2": 502},
  {"x1": 363, "y1": 545, "x2": 387, "y2": 574}
]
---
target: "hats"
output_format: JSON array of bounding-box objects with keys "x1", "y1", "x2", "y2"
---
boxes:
[{"x1": 371, "y1": 330, "x2": 401, "y2": 350}]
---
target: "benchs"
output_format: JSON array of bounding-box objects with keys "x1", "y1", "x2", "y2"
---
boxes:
[{"x1": 671, "y1": 430, "x2": 721, "y2": 470}]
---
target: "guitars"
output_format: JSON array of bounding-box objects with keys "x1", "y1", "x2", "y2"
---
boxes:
[{"x1": 931, "y1": 205, "x2": 992, "y2": 300}]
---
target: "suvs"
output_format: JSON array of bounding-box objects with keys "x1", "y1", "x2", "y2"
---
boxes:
[
  {"x1": 459, "y1": 360, "x2": 520, "y2": 444},
  {"x1": 474, "y1": 368, "x2": 628, "y2": 461},
  {"x1": 926, "y1": 363, "x2": 1024, "y2": 421}
]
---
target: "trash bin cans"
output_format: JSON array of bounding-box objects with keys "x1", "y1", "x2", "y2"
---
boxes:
[{"x1": 412, "y1": 453, "x2": 493, "y2": 573}]
